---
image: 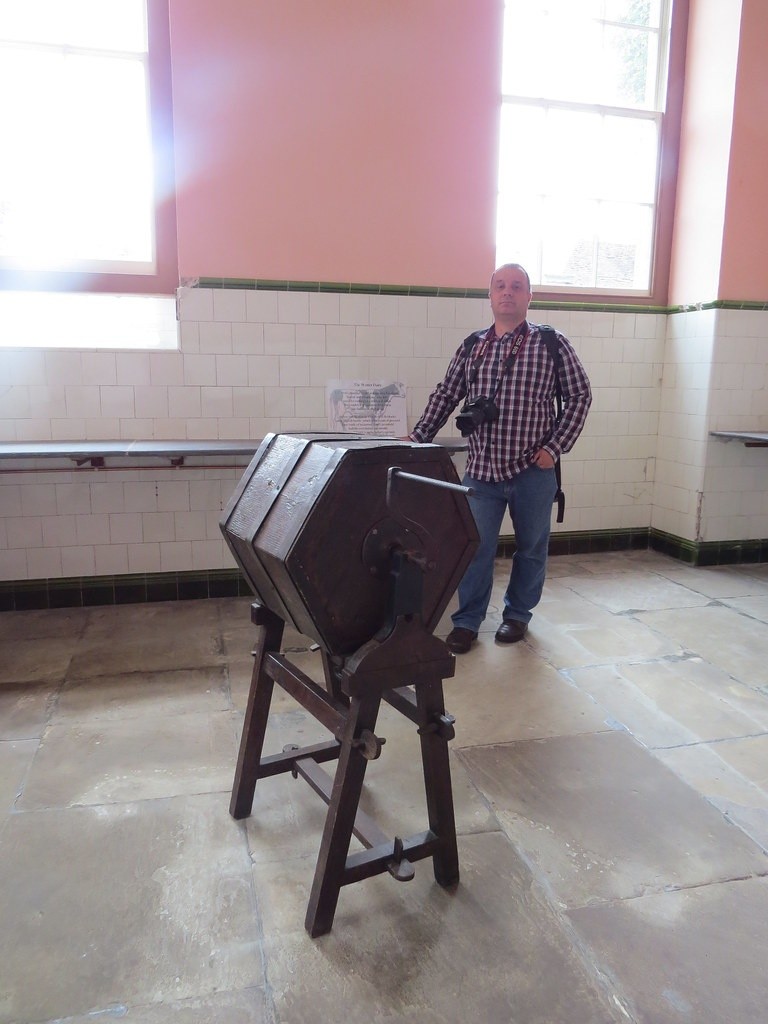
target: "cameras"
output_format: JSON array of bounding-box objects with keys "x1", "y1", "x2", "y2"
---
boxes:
[{"x1": 455, "y1": 395, "x2": 500, "y2": 438}]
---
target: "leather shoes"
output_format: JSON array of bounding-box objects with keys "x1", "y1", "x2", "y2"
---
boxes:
[
  {"x1": 444, "y1": 625, "x2": 479, "y2": 654},
  {"x1": 494, "y1": 618, "x2": 529, "y2": 643}
]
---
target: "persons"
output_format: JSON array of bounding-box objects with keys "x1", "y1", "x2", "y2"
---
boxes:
[{"x1": 398, "y1": 264, "x2": 592, "y2": 654}]
